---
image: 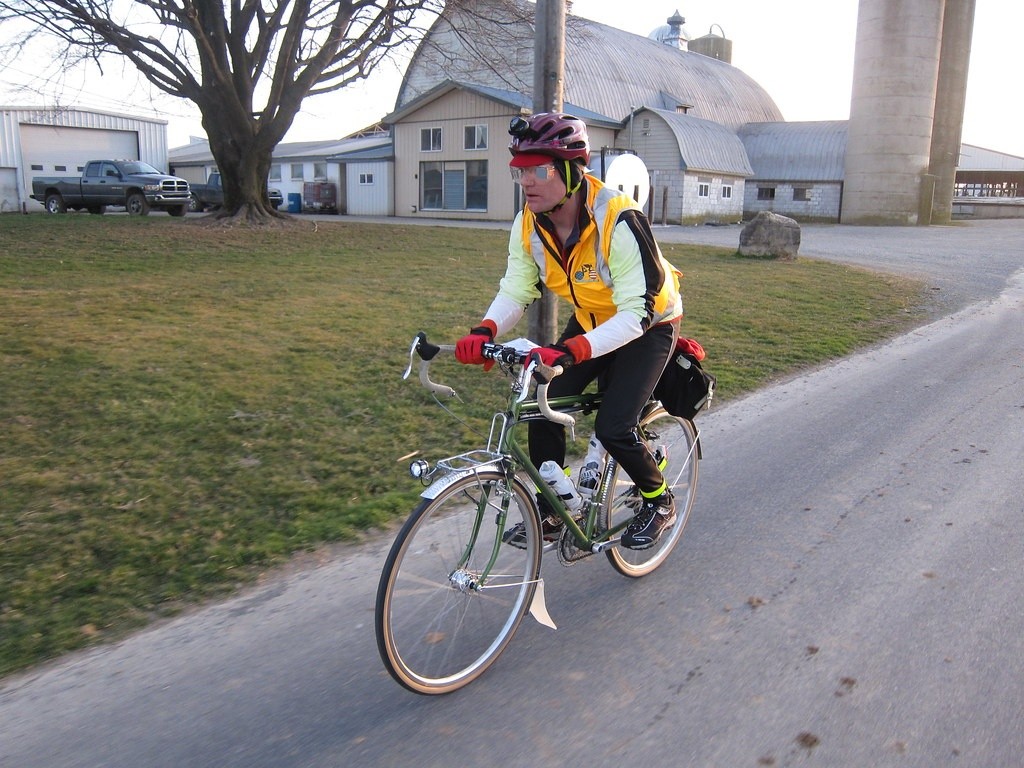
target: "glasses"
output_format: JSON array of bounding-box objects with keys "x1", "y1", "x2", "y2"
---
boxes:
[{"x1": 510, "y1": 165, "x2": 557, "y2": 183}]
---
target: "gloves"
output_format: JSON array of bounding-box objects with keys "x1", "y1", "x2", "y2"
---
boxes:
[
  {"x1": 523, "y1": 343, "x2": 573, "y2": 384},
  {"x1": 455, "y1": 326, "x2": 496, "y2": 371}
]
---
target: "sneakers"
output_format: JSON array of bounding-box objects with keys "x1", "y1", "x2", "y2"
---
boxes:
[
  {"x1": 621, "y1": 495, "x2": 677, "y2": 551},
  {"x1": 502, "y1": 513, "x2": 563, "y2": 549}
]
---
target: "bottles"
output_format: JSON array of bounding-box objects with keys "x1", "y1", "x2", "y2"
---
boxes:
[
  {"x1": 577, "y1": 427, "x2": 607, "y2": 493},
  {"x1": 539, "y1": 461, "x2": 582, "y2": 509}
]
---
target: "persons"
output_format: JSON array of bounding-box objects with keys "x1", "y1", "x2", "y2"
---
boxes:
[{"x1": 456, "y1": 112, "x2": 684, "y2": 551}]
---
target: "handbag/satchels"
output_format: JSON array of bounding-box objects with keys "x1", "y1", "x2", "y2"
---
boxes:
[{"x1": 653, "y1": 348, "x2": 717, "y2": 421}]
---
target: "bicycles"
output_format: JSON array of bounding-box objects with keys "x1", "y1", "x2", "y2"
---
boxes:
[{"x1": 372, "y1": 329, "x2": 705, "y2": 696}]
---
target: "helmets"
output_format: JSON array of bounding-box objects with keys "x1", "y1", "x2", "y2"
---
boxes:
[{"x1": 508, "y1": 112, "x2": 589, "y2": 165}]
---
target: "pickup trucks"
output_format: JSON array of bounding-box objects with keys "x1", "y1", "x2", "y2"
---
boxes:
[
  {"x1": 29, "y1": 157, "x2": 192, "y2": 217},
  {"x1": 186, "y1": 171, "x2": 284, "y2": 214}
]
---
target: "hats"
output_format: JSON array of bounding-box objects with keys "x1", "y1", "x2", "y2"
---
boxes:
[{"x1": 509, "y1": 152, "x2": 555, "y2": 167}]
